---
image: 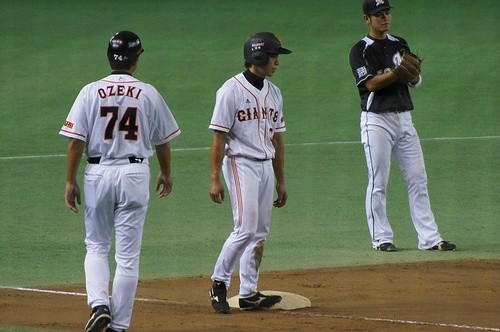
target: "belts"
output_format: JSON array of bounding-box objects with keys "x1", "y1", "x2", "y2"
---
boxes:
[
  {"x1": 87, "y1": 155, "x2": 143, "y2": 164},
  {"x1": 256, "y1": 158, "x2": 267, "y2": 161},
  {"x1": 373, "y1": 108, "x2": 405, "y2": 114}
]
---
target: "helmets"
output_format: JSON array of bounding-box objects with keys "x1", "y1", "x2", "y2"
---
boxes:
[
  {"x1": 243, "y1": 32, "x2": 293, "y2": 67},
  {"x1": 108, "y1": 31, "x2": 145, "y2": 65}
]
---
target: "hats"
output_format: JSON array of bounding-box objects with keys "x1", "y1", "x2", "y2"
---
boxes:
[{"x1": 362, "y1": 0, "x2": 395, "y2": 15}]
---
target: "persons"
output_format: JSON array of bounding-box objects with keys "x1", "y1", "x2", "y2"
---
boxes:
[
  {"x1": 349, "y1": 0, "x2": 456, "y2": 251},
  {"x1": 209, "y1": 32, "x2": 292, "y2": 315},
  {"x1": 60, "y1": 31, "x2": 182, "y2": 332}
]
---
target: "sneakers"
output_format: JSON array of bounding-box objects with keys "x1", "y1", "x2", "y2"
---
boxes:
[
  {"x1": 209, "y1": 280, "x2": 230, "y2": 312},
  {"x1": 238, "y1": 292, "x2": 283, "y2": 310},
  {"x1": 84, "y1": 306, "x2": 113, "y2": 332},
  {"x1": 105, "y1": 327, "x2": 125, "y2": 332},
  {"x1": 426, "y1": 240, "x2": 457, "y2": 251},
  {"x1": 374, "y1": 243, "x2": 397, "y2": 251}
]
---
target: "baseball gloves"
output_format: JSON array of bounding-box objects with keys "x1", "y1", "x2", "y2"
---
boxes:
[{"x1": 391, "y1": 48, "x2": 422, "y2": 84}]
